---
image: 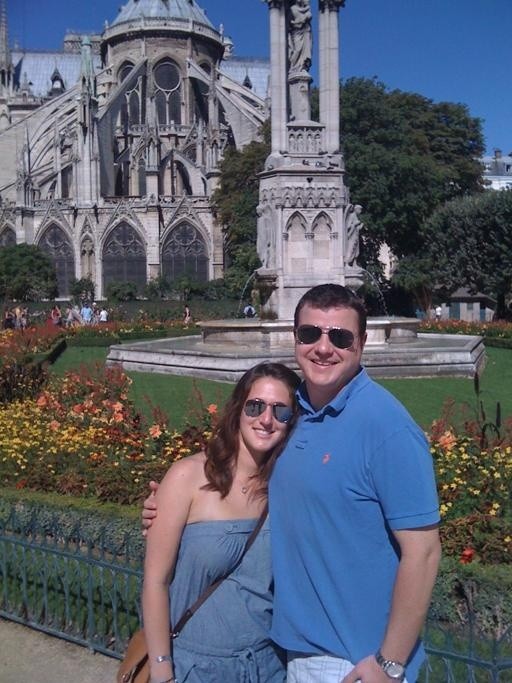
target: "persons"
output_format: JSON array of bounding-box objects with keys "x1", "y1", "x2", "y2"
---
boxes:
[
  {"x1": 142, "y1": 282, "x2": 446, "y2": 682},
  {"x1": 51, "y1": 301, "x2": 110, "y2": 326},
  {"x1": 143, "y1": 362, "x2": 301, "y2": 682},
  {"x1": 2, "y1": 303, "x2": 33, "y2": 330}
]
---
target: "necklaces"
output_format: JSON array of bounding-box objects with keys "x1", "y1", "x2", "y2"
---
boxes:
[{"x1": 239, "y1": 481, "x2": 248, "y2": 494}]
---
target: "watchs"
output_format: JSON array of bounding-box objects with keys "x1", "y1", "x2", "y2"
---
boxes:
[{"x1": 375, "y1": 648, "x2": 405, "y2": 679}]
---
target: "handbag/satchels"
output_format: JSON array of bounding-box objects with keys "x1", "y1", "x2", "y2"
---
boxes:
[{"x1": 116, "y1": 626, "x2": 171, "y2": 683}]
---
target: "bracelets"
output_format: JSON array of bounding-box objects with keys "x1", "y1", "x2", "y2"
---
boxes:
[{"x1": 158, "y1": 656, "x2": 173, "y2": 662}]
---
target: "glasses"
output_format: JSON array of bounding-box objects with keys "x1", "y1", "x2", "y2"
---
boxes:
[
  {"x1": 243, "y1": 398, "x2": 294, "y2": 424},
  {"x1": 295, "y1": 323, "x2": 361, "y2": 349}
]
